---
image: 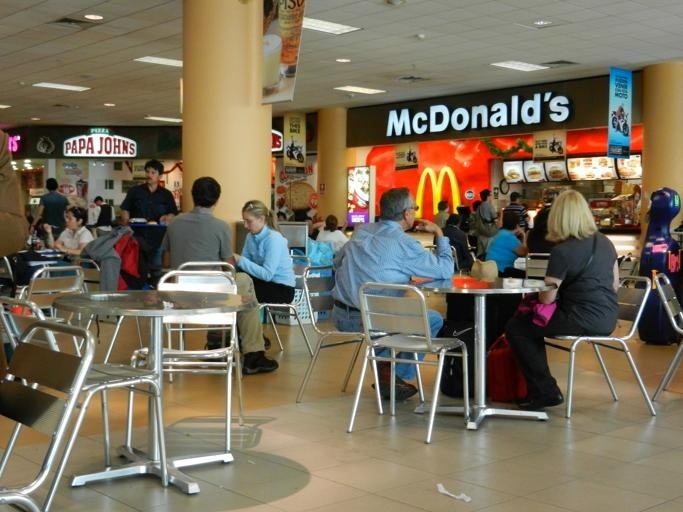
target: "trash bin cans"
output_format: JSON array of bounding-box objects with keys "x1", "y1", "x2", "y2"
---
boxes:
[{"x1": 236, "y1": 221, "x2": 308, "y2": 322}]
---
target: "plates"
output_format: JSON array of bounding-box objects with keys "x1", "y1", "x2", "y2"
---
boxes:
[{"x1": 128, "y1": 221, "x2": 147, "y2": 224}]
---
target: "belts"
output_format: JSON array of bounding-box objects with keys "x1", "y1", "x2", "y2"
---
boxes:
[{"x1": 334, "y1": 299, "x2": 361, "y2": 313}]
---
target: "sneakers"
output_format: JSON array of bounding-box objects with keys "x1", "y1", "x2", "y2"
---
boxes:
[
  {"x1": 206, "y1": 333, "x2": 271, "y2": 354},
  {"x1": 241, "y1": 350, "x2": 279, "y2": 375},
  {"x1": 371, "y1": 373, "x2": 418, "y2": 402},
  {"x1": 518, "y1": 377, "x2": 564, "y2": 409}
]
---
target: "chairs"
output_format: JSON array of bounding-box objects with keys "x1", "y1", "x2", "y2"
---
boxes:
[
  {"x1": 0, "y1": 250, "x2": 240, "y2": 511},
  {"x1": 255, "y1": 255, "x2": 311, "y2": 360},
  {"x1": 652, "y1": 272, "x2": 682, "y2": 402},
  {"x1": 295, "y1": 245, "x2": 556, "y2": 443},
  {"x1": 544, "y1": 275, "x2": 657, "y2": 417}
]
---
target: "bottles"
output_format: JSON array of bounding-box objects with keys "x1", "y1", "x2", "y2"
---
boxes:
[{"x1": 31, "y1": 231, "x2": 37, "y2": 243}]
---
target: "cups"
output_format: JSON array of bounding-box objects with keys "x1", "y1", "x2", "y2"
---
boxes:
[{"x1": 76, "y1": 181, "x2": 88, "y2": 198}]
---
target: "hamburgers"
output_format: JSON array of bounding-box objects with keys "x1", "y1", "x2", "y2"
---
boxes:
[
  {"x1": 507, "y1": 169, "x2": 521, "y2": 181},
  {"x1": 548, "y1": 166, "x2": 562, "y2": 178},
  {"x1": 527, "y1": 167, "x2": 541, "y2": 180}
]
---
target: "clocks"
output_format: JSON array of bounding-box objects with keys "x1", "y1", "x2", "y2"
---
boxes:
[{"x1": 499, "y1": 178, "x2": 510, "y2": 195}]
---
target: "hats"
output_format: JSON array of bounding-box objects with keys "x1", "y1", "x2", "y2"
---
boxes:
[{"x1": 510, "y1": 191, "x2": 522, "y2": 199}]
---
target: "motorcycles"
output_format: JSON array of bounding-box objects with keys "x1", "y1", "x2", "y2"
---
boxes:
[
  {"x1": 549, "y1": 140, "x2": 563, "y2": 154},
  {"x1": 610, "y1": 110, "x2": 628, "y2": 136},
  {"x1": 406, "y1": 151, "x2": 417, "y2": 163},
  {"x1": 286, "y1": 145, "x2": 304, "y2": 163}
]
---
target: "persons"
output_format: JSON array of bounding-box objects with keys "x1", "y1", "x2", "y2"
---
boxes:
[
  {"x1": 433, "y1": 188, "x2": 557, "y2": 276},
  {"x1": 232, "y1": 199, "x2": 297, "y2": 352},
  {"x1": 278, "y1": 210, "x2": 350, "y2": 260},
  {"x1": 289, "y1": 135, "x2": 296, "y2": 160},
  {"x1": 0, "y1": 129, "x2": 30, "y2": 258},
  {"x1": 330, "y1": 185, "x2": 455, "y2": 401},
  {"x1": 615, "y1": 103, "x2": 625, "y2": 131},
  {"x1": 634, "y1": 192, "x2": 641, "y2": 208},
  {"x1": 505, "y1": 189, "x2": 620, "y2": 410},
  {"x1": 25, "y1": 158, "x2": 178, "y2": 289},
  {"x1": 162, "y1": 175, "x2": 279, "y2": 376}
]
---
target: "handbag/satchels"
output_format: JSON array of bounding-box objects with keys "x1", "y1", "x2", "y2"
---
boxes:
[
  {"x1": 478, "y1": 215, "x2": 498, "y2": 237},
  {"x1": 440, "y1": 326, "x2": 475, "y2": 398},
  {"x1": 485, "y1": 333, "x2": 527, "y2": 402}
]
